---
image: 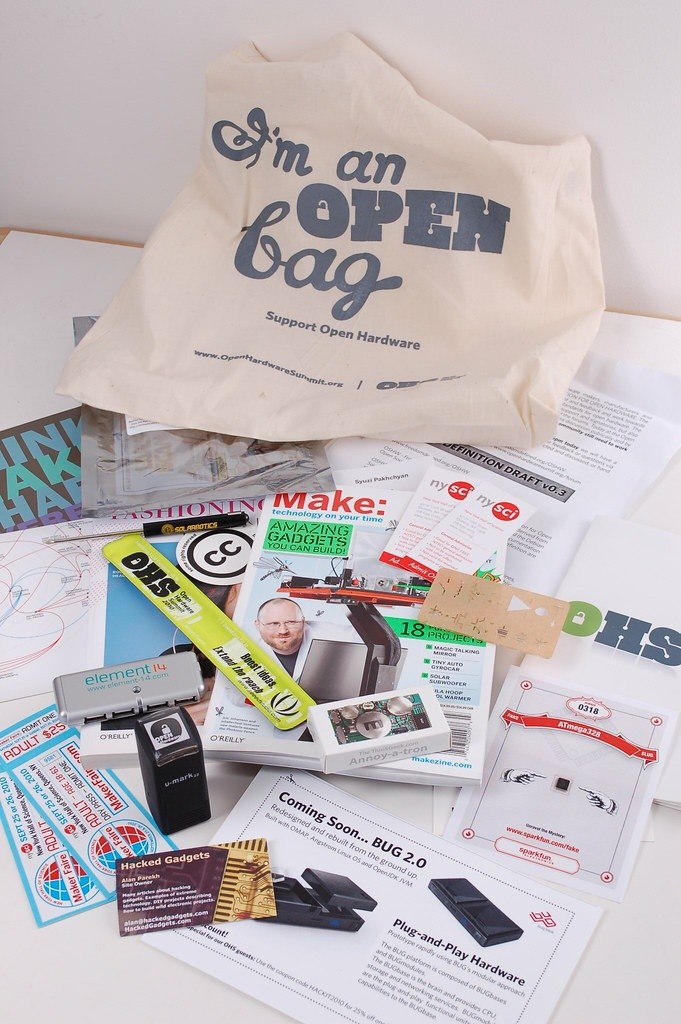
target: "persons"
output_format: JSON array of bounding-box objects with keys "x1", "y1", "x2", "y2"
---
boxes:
[{"x1": 253, "y1": 598, "x2": 352, "y2": 684}]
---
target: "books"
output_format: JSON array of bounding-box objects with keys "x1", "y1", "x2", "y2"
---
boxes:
[{"x1": 80, "y1": 451, "x2": 507, "y2": 788}]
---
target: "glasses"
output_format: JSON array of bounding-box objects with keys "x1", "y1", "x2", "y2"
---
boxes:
[{"x1": 258, "y1": 619, "x2": 303, "y2": 628}]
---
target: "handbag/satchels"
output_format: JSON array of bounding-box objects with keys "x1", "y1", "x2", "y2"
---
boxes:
[{"x1": 57, "y1": 28, "x2": 606, "y2": 451}]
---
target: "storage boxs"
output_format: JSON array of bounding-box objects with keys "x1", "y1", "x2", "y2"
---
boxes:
[
  {"x1": 305, "y1": 681, "x2": 453, "y2": 775},
  {"x1": 132, "y1": 705, "x2": 213, "y2": 838}
]
